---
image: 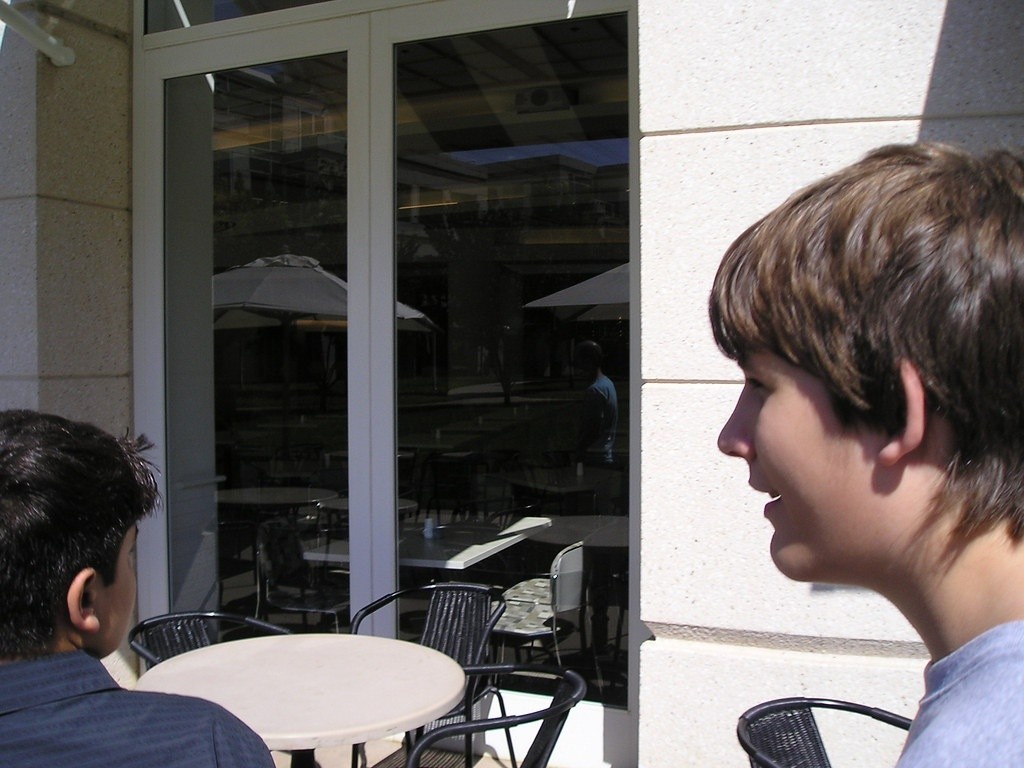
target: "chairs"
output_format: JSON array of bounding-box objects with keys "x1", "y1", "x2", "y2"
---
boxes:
[
  {"x1": 368, "y1": 664, "x2": 587, "y2": 768},
  {"x1": 734, "y1": 696, "x2": 913, "y2": 768},
  {"x1": 347, "y1": 580, "x2": 520, "y2": 768},
  {"x1": 254, "y1": 517, "x2": 350, "y2": 635},
  {"x1": 501, "y1": 519, "x2": 619, "y2": 667},
  {"x1": 127, "y1": 610, "x2": 293, "y2": 672},
  {"x1": 485, "y1": 535, "x2": 597, "y2": 669}
]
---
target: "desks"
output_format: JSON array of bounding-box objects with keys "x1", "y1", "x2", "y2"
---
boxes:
[
  {"x1": 214, "y1": 371, "x2": 629, "y2": 666},
  {"x1": 130, "y1": 633, "x2": 466, "y2": 768}
]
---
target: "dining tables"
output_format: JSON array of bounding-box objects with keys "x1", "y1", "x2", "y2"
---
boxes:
[{"x1": 302, "y1": 511, "x2": 553, "y2": 571}]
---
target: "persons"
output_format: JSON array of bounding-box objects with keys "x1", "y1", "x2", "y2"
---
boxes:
[
  {"x1": 559, "y1": 338, "x2": 618, "y2": 518},
  {"x1": 1, "y1": 404, "x2": 279, "y2": 766},
  {"x1": 707, "y1": 138, "x2": 1024, "y2": 768}
]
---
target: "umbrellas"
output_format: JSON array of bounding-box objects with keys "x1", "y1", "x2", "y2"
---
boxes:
[
  {"x1": 208, "y1": 253, "x2": 442, "y2": 332},
  {"x1": 520, "y1": 256, "x2": 630, "y2": 322}
]
